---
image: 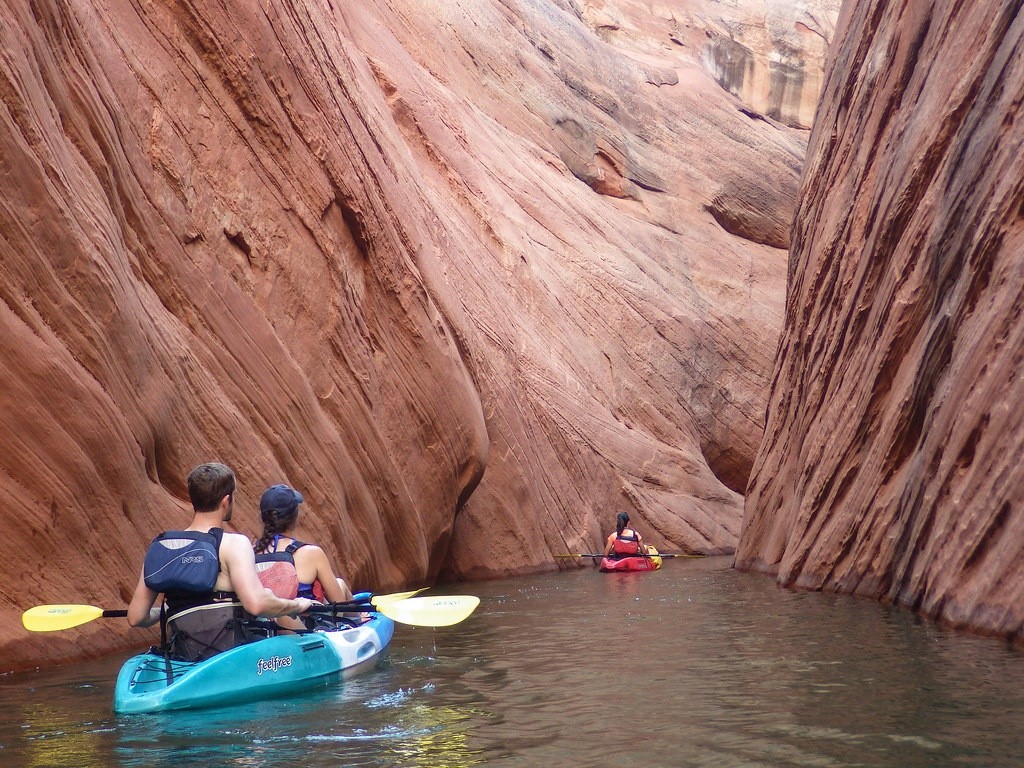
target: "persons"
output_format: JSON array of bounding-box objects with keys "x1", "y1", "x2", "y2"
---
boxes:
[
  {"x1": 603, "y1": 511, "x2": 648, "y2": 561},
  {"x1": 127, "y1": 463, "x2": 371, "y2": 662}
]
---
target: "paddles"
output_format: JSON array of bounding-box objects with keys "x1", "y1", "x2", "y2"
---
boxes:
[
  {"x1": 551, "y1": 554, "x2": 707, "y2": 559},
  {"x1": 21, "y1": 587, "x2": 482, "y2": 633}
]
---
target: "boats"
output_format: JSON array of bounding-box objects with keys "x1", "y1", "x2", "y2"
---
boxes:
[
  {"x1": 112, "y1": 592, "x2": 394, "y2": 715},
  {"x1": 598, "y1": 545, "x2": 663, "y2": 573}
]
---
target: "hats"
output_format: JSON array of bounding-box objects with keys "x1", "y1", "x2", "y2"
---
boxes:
[{"x1": 259, "y1": 484, "x2": 304, "y2": 520}]
